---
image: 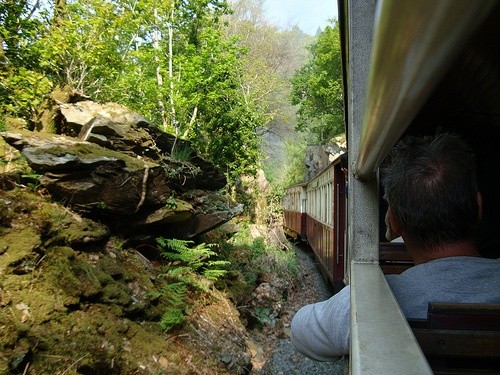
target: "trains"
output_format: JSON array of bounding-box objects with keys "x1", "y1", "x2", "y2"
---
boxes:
[{"x1": 280, "y1": 0, "x2": 500, "y2": 375}]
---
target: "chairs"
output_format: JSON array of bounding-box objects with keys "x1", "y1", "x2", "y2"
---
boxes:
[
  {"x1": 379, "y1": 242, "x2": 415, "y2": 275},
  {"x1": 407, "y1": 302, "x2": 500, "y2": 375}
]
